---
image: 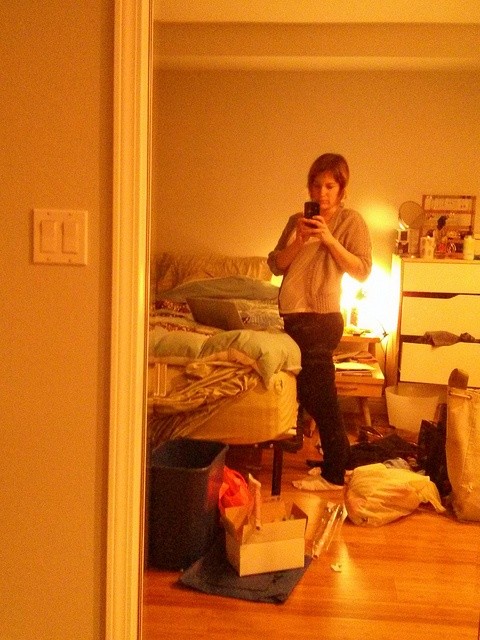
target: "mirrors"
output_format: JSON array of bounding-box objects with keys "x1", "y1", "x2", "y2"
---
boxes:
[{"x1": 102, "y1": 0, "x2": 480, "y2": 640}]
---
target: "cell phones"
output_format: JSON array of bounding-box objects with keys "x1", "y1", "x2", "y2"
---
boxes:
[{"x1": 303, "y1": 202, "x2": 321, "y2": 222}]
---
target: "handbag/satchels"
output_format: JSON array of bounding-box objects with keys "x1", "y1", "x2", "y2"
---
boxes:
[{"x1": 446, "y1": 368, "x2": 480, "y2": 521}]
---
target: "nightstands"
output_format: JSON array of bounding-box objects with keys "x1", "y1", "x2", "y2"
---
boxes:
[{"x1": 306, "y1": 322, "x2": 387, "y2": 442}]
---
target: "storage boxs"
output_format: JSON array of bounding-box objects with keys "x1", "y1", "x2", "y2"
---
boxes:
[{"x1": 222, "y1": 500, "x2": 309, "y2": 577}]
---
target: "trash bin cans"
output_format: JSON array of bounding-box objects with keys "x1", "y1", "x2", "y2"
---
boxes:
[{"x1": 149, "y1": 437, "x2": 229, "y2": 571}]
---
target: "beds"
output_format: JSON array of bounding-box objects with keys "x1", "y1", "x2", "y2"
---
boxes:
[{"x1": 146, "y1": 299, "x2": 300, "y2": 495}]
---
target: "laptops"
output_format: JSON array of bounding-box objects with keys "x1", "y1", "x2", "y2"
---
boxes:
[{"x1": 185, "y1": 296, "x2": 268, "y2": 331}]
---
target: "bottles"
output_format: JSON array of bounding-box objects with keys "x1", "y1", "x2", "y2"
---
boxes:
[{"x1": 462, "y1": 231, "x2": 475, "y2": 262}]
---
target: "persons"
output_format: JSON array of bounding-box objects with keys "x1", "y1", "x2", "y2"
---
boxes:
[{"x1": 266, "y1": 153, "x2": 373, "y2": 491}]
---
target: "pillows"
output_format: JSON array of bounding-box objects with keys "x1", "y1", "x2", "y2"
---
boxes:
[
  {"x1": 157, "y1": 329, "x2": 302, "y2": 390},
  {"x1": 160, "y1": 276, "x2": 280, "y2": 305},
  {"x1": 157, "y1": 247, "x2": 274, "y2": 299}
]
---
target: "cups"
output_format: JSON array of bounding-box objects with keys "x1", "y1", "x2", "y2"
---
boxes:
[{"x1": 419, "y1": 235, "x2": 436, "y2": 260}]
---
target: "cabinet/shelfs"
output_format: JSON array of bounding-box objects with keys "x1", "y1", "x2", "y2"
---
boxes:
[{"x1": 391, "y1": 239, "x2": 480, "y2": 389}]
